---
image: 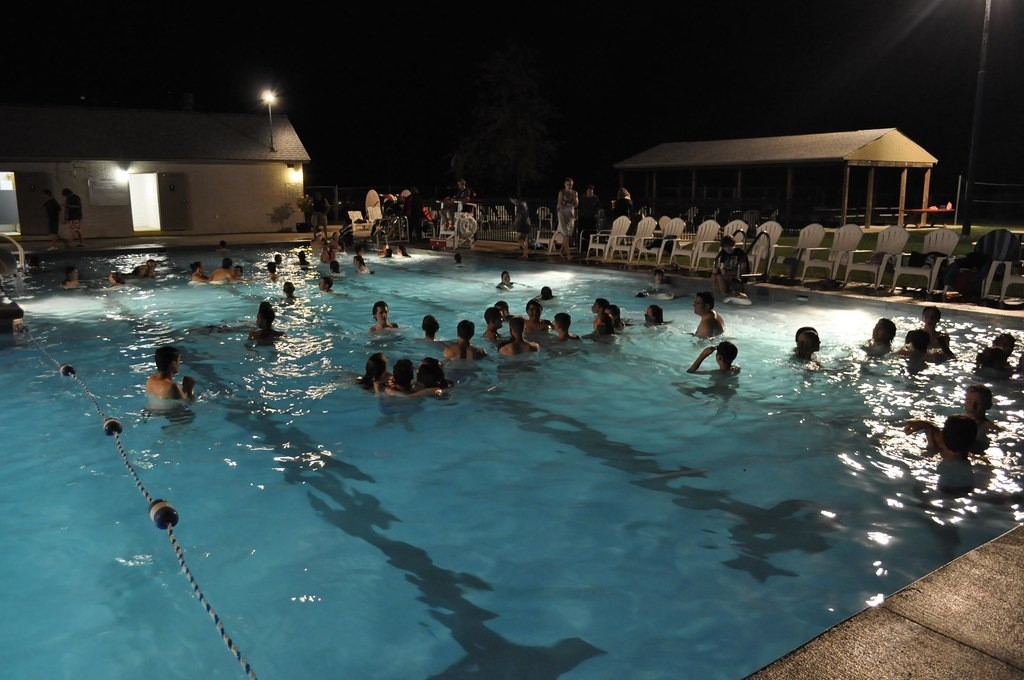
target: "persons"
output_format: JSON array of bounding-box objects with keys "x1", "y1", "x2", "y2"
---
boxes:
[
  {"x1": 688, "y1": 341, "x2": 738, "y2": 371},
  {"x1": 147, "y1": 346, "x2": 194, "y2": 399},
  {"x1": 711, "y1": 235, "x2": 749, "y2": 296},
  {"x1": 369, "y1": 302, "x2": 399, "y2": 331},
  {"x1": 905, "y1": 384, "x2": 999, "y2": 458},
  {"x1": 247, "y1": 303, "x2": 286, "y2": 341},
  {"x1": 509, "y1": 177, "x2": 645, "y2": 262},
  {"x1": 422, "y1": 315, "x2": 439, "y2": 341},
  {"x1": 654, "y1": 268, "x2": 664, "y2": 283},
  {"x1": 975, "y1": 333, "x2": 1024, "y2": 379},
  {"x1": 795, "y1": 327, "x2": 820, "y2": 360},
  {"x1": 444, "y1": 299, "x2": 621, "y2": 361},
  {"x1": 40, "y1": 186, "x2": 244, "y2": 288},
  {"x1": 867, "y1": 306, "x2": 951, "y2": 367},
  {"x1": 541, "y1": 287, "x2": 553, "y2": 299},
  {"x1": 357, "y1": 352, "x2": 453, "y2": 396},
  {"x1": 497, "y1": 271, "x2": 513, "y2": 290},
  {"x1": 646, "y1": 305, "x2": 664, "y2": 325},
  {"x1": 692, "y1": 292, "x2": 725, "y2": 337},
  {"x1": 267, "y1": 179, "x2": 470, "y2": 296}
]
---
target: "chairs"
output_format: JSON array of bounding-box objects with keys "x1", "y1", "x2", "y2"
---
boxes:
[{"x1": 422, "y1": 202, "x2": 1024, "y2": 302}]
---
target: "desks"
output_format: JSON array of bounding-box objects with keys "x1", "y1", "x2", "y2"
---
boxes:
[{"x1": 901, "y1": 210, "x2": 955, "y2": 229}]
